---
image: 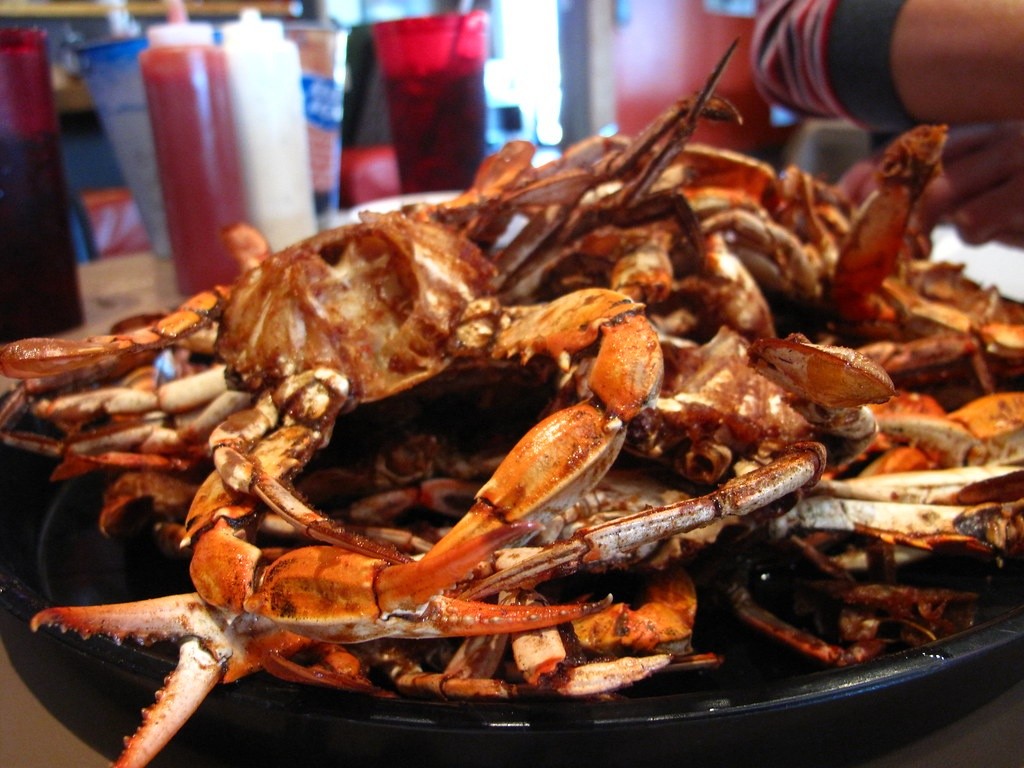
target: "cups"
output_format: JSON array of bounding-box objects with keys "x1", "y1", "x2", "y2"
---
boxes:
[
  {"x1": 373, "y1": 9, "x2": 487, "y2": 196},
  {"x1": 1, "y1": 25, "x2": 87, "y2": 342},
  {"x1": 279, "y1": 20, "x2": 338, "y2": 223},
  {"x1": 77, "y1": 33, "x2": 170, "y2": 263}
]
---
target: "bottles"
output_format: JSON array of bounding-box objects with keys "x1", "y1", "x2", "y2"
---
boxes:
[
  {"x1": 222, "y1": 7, "x2": 321, "y2": 255},
  {"x1": 142, "y1": 0, "x2": 248, "y2": 294}
]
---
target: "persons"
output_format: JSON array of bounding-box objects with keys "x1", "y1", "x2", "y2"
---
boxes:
[{"x1": 750, "y1": 0, "x2": 1024, "y2": 249}]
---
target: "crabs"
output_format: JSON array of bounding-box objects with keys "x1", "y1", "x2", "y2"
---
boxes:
[{"x1": 1, "y1": 36, "x2": 1024, "y2": 767}]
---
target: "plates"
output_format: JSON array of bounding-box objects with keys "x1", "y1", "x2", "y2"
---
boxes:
[{"x1": 1, "y1": 394, "x2": 1024, "y2": 768}]
